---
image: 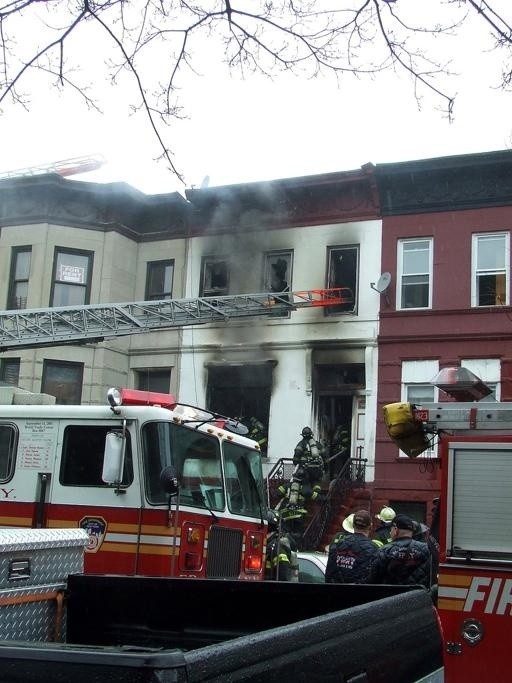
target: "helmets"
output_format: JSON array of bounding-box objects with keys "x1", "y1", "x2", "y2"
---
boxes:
[
  {"x1": 268, "y1": 510, "x2": 280, "y2": 524},
  {"x1": 300, "y1": 427, "x2": 314, "y2": 435},
  {"x1": 342, "y1": 513, "x2": 355, "y2": 533},
  {"x1": 377, "y1": 507, "x2": 396, "y2": 523}
]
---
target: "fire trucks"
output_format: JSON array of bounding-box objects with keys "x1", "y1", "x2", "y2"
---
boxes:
[
  {"x1": 0, "y1": 387, "x2": 270, "y2": 577},
  {"x1": 386, "y1": 366, "x2": 512, "y2": 682}
]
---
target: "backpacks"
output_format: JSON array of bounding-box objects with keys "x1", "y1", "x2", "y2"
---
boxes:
[{"x1": 301, "y1": 439, "x2": 325, "y2": 469}]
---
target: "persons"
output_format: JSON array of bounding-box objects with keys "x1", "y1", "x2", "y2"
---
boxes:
[
  {"x1": 238, "y1": 413, "x2": 267, "y2": 457},
  {"x1": 325, "y1": 507, "x2": 439, "y2": 609},
  {"x1": 293, "y1": 427, "x2": 328, "y2": 493},
  {"x1": 265, "y1": 469, "x2": 324, "y2": 581},
  {"x1": 332, "y1": 424, "x2": 350, "y2": 450}
]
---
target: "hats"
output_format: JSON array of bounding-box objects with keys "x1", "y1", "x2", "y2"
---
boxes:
[
  {"x1": 354, "y1": 510, "x2": 372, "y2": 529},
  {"x1": 391, "y1": 514, "x2": 413, "y2": 530}
]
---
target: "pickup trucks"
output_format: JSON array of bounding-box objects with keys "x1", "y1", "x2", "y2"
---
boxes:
[{"x1": 0, "y1": 528, "x2": 445, "y2": 681}]
